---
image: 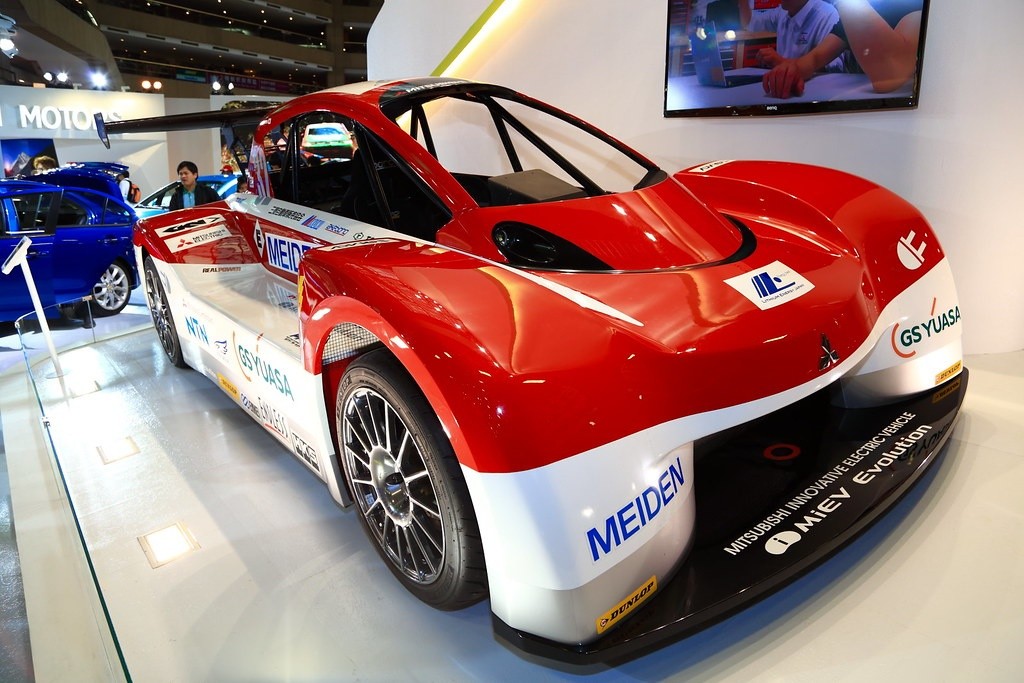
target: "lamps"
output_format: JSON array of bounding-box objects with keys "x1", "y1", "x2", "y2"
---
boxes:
[
  {"x1": 43, "y1": 72, "x2": 56, "y2": 84},
  {"x1": 0, "y1": 35, "x2": 19, "y2": 59}
]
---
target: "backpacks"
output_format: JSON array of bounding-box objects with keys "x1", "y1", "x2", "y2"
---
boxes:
[{"x1": 126, "y1": 179, "x2": 140, "y2": 204}]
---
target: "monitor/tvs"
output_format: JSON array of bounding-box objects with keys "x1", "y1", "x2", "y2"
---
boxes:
[{"x1": 663, "y1": 0, "x2": 930, "y2": 119}]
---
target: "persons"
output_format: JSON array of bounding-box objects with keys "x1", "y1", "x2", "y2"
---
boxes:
[
  {"x1": 116, "y1": 170, "x2": 132, "y2": 200},
  {"x1": 220, "y1": 164, "x2": 233, "y2": 174},
  {"x1": 737, "y1": 0, "x2": 847, "y2": 72},
  {"x1": 32, "y1": 156, "x2": 56, "y2": 175},
  {"x1": 168, "y1": 161, "x2": 222, "y2": 211},
  {"x1": 761, "y1": 0, "x2": 923, "y2": 98}
]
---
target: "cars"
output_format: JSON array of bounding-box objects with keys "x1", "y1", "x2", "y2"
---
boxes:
[
  {"x1": 0, "y1": 180, "x2": 140, "y2": 324},
  {"x1": 130, "y1": 173, "x2": 244, "y2": 222},
  {"x1": 134, "y1": 78, "x2": 973, "y2": 664}
]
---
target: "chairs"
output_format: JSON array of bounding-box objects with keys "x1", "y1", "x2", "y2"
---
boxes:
[{"x1": 348, "y1": 146, "x2": 433, "y2": 240}]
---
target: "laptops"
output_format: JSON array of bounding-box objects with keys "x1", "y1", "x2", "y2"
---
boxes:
[{"x1": 688, "y1": 21, "x2": 765, "y2": 88}]
